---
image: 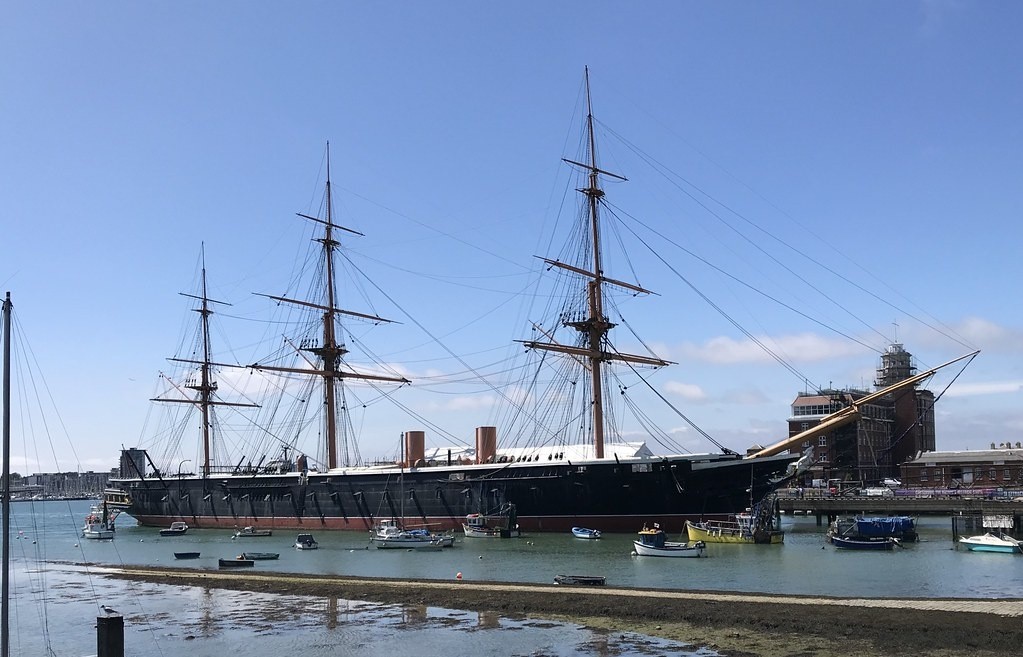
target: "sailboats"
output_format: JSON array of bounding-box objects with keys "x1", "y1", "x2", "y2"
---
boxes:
[{"x1": 102, "y1": 63, "x2": 983, "y2": 535}]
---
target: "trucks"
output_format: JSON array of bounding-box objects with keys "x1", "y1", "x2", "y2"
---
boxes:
[{"x1": 812, "y1": 479, "x2": 827, "y2": 488}]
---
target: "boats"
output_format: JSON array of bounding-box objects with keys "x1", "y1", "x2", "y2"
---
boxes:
[
  {"x1": 826, "y1": 514, "x2": 920, "y2": 550},
  {"x1": 632, "y1": 522, "x2": 708, "y2": 558},
  {"x1": 369, "y1": 520, "x2": 455, "y2": 552},
  {"x1": 572, "y1": 526, "x2": 602, "y2": 539},
  {"x1": 82, "y1": 503, "x2": 117, "y2": 540},
  {"x1": 461, "y1": 502, "x2": 521, "y2": 538},
  {"x1": 295, "y1": 534, "x2": 318, "y2": 549},
  {"x1": 159, "y1": 522, "x2": 189, "y2": 536},
  {"x1": 685, "y1": 508, "x2": 784, "y2": 544},
  {"x1": 958, "y1": 532, "x2": 1023, "y2": 554},
  {"x1": 234, "y1": 523, "x2": 272, "y2": 536}
]
